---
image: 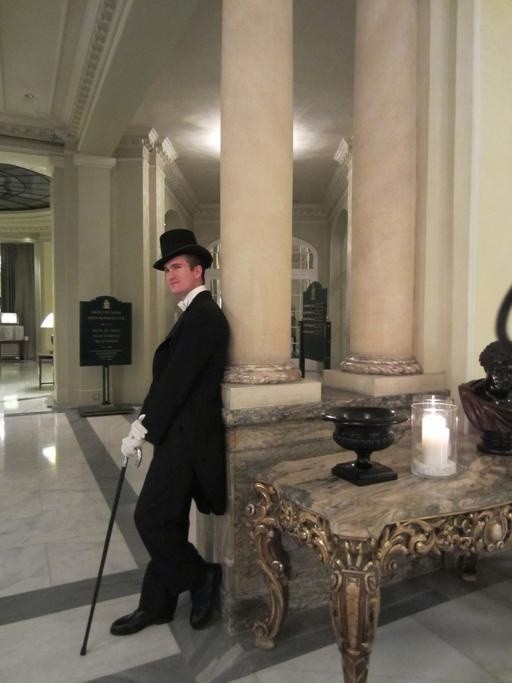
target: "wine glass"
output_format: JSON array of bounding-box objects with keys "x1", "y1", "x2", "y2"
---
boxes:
[{"x1": 321, "y1": 405, "x2": 406, "y2": 487}]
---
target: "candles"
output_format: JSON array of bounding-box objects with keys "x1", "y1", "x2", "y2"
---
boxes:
[{"x1": 422, "y1": 414, "x2": 449, "y2": 462}]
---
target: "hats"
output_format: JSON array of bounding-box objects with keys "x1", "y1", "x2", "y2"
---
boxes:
[{"x1": 152, "y1": 229, "x2": 213, "y2": 270}]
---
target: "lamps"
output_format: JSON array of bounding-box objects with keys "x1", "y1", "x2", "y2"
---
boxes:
[{"x1": 38, "y1": 311, "x2": 54, "y2": 353}]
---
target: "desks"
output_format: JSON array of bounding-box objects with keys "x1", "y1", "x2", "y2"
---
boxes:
[
  {"x1": 0, "y1": 339, "x2": 32, "y2": 362},
  {"x1": 37, "y1": 354, "x2": 55, "y2": 389},
  {"x1": 243, "y1": 434, "x2": 511, "y2": 682}
]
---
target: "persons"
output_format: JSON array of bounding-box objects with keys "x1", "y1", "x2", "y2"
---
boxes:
[{"x1": 109, "y1": 228, "x2": 229, "y2": 634}]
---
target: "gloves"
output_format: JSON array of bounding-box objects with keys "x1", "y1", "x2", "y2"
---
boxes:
[{"x1": 120, "y1": 414, "x2": 149, "y2": 457}]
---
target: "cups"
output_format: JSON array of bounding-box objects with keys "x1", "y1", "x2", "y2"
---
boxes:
[{"x1": 410, "y1": 393, "x2": 460, "y2": 481}]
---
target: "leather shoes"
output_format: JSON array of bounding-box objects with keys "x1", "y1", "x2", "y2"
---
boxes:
[
  {"x1": 189, "y1": 562, "x2": 222, "y2": 629},
  {"x1": 110, "y1": 608, "x2": 174, "y2": 634}
]
